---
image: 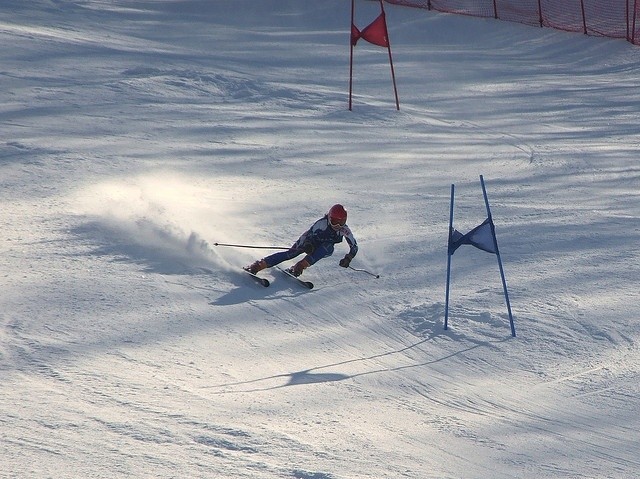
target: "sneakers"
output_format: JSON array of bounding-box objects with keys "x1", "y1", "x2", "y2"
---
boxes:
[
  {"x1": 243, "y1": 264, "x2": 261, "y2": 274},
  {"x1": 289, "y1": 264, "x2": 303, "y2": 273}
]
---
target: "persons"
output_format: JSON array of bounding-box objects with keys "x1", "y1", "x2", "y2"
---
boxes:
[{"x1": 246, "y1": 202, "x2": 359, "y2": 291}]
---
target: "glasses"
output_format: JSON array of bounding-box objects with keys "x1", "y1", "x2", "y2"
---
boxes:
[{"x1": 329, "y1": 218, "x2": 346, "y2": 228}]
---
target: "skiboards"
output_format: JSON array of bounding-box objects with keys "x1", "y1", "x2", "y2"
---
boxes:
[{"x1": 242, "y1": 265, "x2": 314, "y2": 289}]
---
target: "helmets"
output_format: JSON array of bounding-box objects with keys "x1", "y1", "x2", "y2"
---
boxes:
[{"x1": 328, "y1": 204, "x2": 347, "y2": 220}]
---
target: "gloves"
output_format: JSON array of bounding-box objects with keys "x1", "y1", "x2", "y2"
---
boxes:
[
  {"x1": 303, "y1": 239, "x2": 314, "y2": 256},
  {"x1": 339, "y1": 257, "x2": 351, "y2": 268}
]
